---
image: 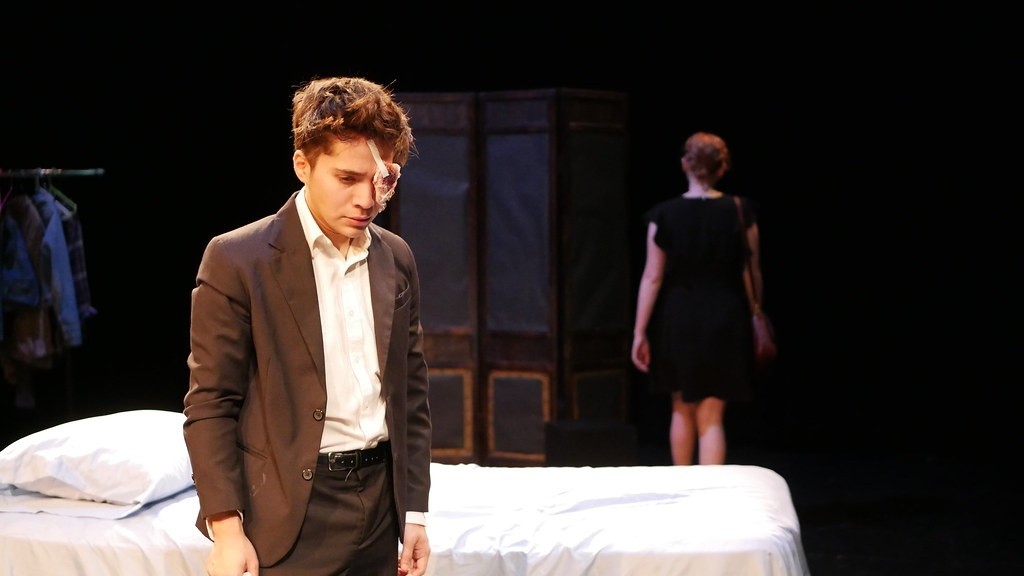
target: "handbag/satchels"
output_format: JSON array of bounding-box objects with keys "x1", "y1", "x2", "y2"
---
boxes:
[{"x1": 744, "y1": 302, "x2": 775, "y2": 364}]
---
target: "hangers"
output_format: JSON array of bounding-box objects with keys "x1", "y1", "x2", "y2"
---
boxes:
[{"x1": 0, "y1": 167, "x2": 59, "y2": 193}]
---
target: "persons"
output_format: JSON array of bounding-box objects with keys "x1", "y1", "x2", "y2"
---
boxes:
[
  {"x1": 631, "y1": 132, "x2": 773, "y2": 465},
  {"x1": 183, "y1": 76, "x2": 435, "y2": 575}
]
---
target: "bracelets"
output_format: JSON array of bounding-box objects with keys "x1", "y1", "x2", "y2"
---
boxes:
[{"x1": 750, "y1": 295, "x2": 763, "y2": 315}]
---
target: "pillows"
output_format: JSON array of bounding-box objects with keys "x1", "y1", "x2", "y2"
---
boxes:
[{"x1": 1, "y1": 410, "x2": 194, "y2": 521}]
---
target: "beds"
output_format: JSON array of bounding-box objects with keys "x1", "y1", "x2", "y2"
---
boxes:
[{"x1": 1, "y1": 463, "x2": 810, "y2": 576}]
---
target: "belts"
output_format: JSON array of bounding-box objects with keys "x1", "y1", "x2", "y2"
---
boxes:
[{"x1": 317, "y1": 443, "x2": 392, "y2": 467}]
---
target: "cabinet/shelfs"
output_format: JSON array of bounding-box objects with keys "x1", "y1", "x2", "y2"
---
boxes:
[{"x1": 390, "y1": 90, "x2": 638, "y2": 466}]
